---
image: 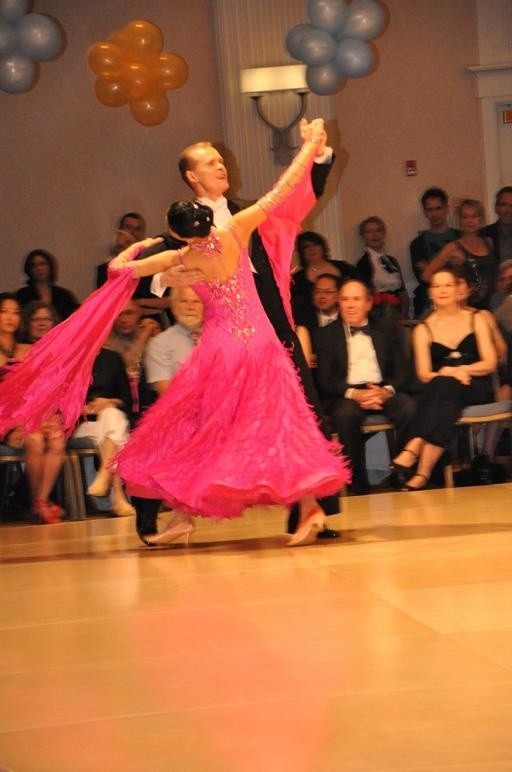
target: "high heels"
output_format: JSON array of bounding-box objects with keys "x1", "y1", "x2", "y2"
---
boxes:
[
  {"x1": 142, "y1": 521, "x2": 195, "y2": 548},
  {"x1": 285, "y1": 509, "x2": 327, "y2": 547}
]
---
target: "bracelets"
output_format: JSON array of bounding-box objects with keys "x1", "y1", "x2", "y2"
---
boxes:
[{"x1": 284, "y1": 149, "x2": 310, "y2": 186}]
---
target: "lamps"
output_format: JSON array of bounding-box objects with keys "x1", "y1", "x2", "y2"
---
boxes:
[{"x1": 240, "y1": 64, "x2": 310, "y2": 165}]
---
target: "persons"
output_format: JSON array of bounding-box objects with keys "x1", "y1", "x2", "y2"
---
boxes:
[
  {"x1": 137, "y1": 119, "x2": 339, "y2": 547},
  {"x1": 0, "y1": 185, "x2": 511, "y2": 527},
  {"x1": 107, "y1": 117, "x2": 353, "y2": 546}
]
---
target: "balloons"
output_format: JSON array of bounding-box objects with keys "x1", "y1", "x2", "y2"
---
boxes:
[
  {"x1": 86, "y1": 17, "x2": 184, "y2": 129},
  {"x1": 0, "y1": 0, "x2": 66, "y2": 97},
  {"x1": 287, "y1": 0, "x2": 392, "y2": 96}
]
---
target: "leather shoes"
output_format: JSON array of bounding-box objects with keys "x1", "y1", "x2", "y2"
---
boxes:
[
  {"x1": 130, "y1": 495, "x2": 159, "y2": 546},
  {"x1": 285, "y1": 516, "x2": 341, "y2": 540}
]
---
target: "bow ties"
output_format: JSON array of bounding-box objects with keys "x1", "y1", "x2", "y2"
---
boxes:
[{"x1": 347, "y1": 324, "x2": 373, "y2": 337}]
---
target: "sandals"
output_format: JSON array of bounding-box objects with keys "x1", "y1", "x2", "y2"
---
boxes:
[
  {"x1": 402, "y1": 470, "x2": 431, "y2": 493},
  {"x1": 29, "y1": 496, "x2": 67, "y2": 525},
  {"x1": 388, "y1": 447, "x2": 418, "y2": 475}
]
